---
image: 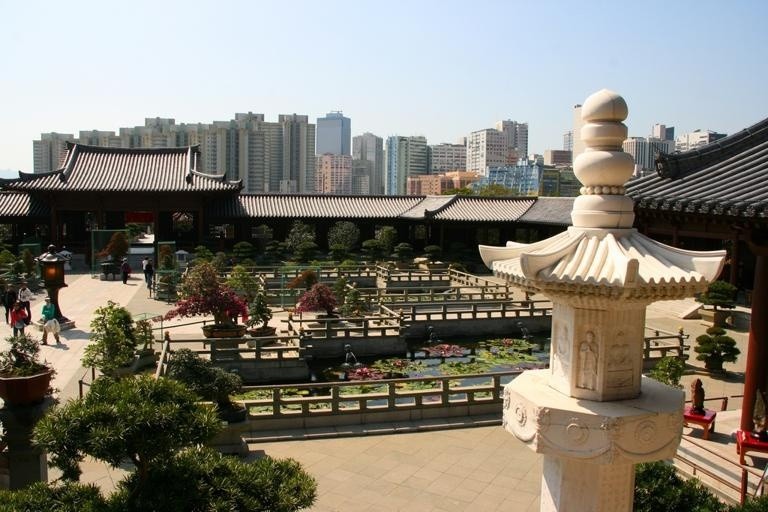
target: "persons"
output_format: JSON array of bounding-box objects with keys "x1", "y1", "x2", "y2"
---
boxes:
[
  {"x1": 141, "y1": 255, "x2": 149, "y2": 283},
  {"x1": 0, "y1": 281, "x2": 32, "y2": 339},
  {"x1": 605, "y1": 329, "x2": 633, "y2": 389},
  {"x1": 119, "y1": 257, "x2": 132, "y2": 285},
  {"x1": 577, "y1": 328, "x2": 600, "y2": 391},
  {"x1": 145, "y1": 258, "x2": 154, "y2": 290},
  {"x1": 39, "y1": 295, "x2": 62, "y2": 346},
  {"x1": 552, "y1": 325, "x2": 571, "y2": 380}
]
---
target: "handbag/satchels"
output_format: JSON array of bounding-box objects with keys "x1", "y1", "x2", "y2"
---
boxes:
[{"x1": 15, "y1": 320, "x2": 24, "y2": 329}]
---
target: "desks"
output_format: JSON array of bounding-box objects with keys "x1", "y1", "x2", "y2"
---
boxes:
[
  {"x1": 736, "y1": 431, "x2": 768, "y2": 465},
  {"x1": 683, "y1": 406, "x2": 716, "y2": 440}
]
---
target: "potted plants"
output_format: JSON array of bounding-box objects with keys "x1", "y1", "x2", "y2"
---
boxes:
[
  {"x1": 693, "y1": 328, "x2": 740, "y2": 369},
  {"x1": 0, "y1": 220, "x2": 50, "y2": 307},
  {"x1": 80, "y1": 221, "x2": 472, "y2": 457},
  {"x1": 0, "y1": 329, "x2": 62, "y2": 407},
  {"x1": 695, "y1": 280, "x2": 739, "y2": 328}
]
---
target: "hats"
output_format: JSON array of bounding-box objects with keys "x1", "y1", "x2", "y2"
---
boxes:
[
  {"x1": 45, "y1": 296, "x2": 51, "y2": 300},
  {"x1": 13, "y1": 301, "x2": 23, "y2": 306}
]
